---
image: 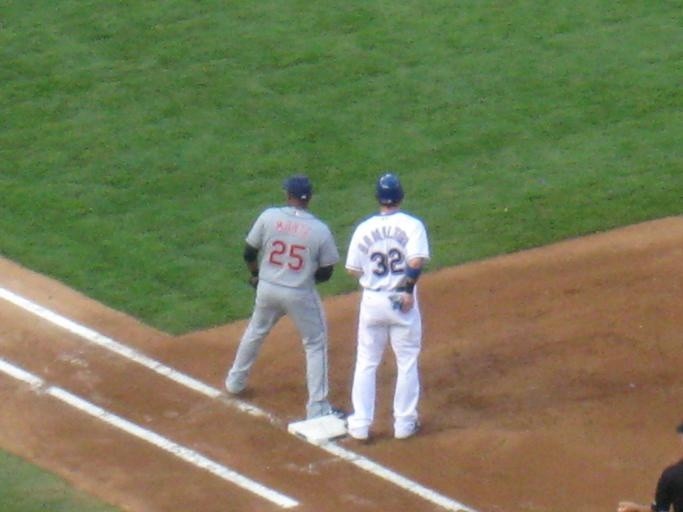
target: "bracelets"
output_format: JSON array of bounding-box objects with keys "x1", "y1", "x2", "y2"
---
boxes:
[{"x1": 404, "y1": 263, "x2": 421, "y2": 279}]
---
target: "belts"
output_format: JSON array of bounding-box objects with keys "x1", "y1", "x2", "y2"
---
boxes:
[{"x1": 376, "y1": 287, "x2": 405, "y2": 292}]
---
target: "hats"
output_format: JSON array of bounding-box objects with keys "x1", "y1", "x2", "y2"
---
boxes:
[
  {"x1": 282, "y1": 173, "x2": 312, "y2": 201},
  {"x1": 375, "y1": 173, "x2": 404, "y2": 205}
]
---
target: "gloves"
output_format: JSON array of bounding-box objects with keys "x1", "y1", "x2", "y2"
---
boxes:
[{"x1": 388, "y1": 294, "x2": 402, "y2": 310}]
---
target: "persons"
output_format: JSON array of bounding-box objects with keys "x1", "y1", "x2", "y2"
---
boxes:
[
  {"x1": 224, "y1": 176, "x2": 346, "y2": 422},
  {"x1": 345, "y1": 174, "x2": 431, "y2": 440},
  {"x1": 615, "y1": 422, "x2": 683, "y2": 512}
]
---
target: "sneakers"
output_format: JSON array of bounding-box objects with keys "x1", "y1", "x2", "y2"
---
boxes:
[
  {"x1": 348, "y1": 423, "x2": 369, "y2": 440},
  {"x1": 330, "y1": 409, "x2": 345, "y2": 418},
  {"x1": 394, "y1": 419, "x2": 422, "y2": 440}
]
